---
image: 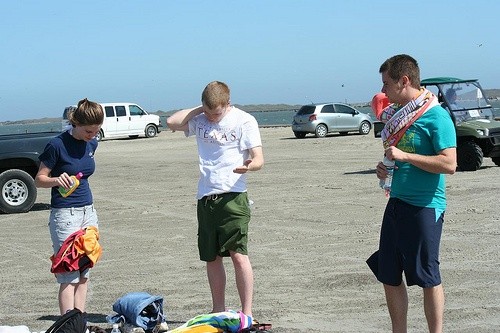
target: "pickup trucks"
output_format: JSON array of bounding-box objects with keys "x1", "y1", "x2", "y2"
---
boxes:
[{"x1": 0, "y1": 132, "x2": 68, "y2": 212}]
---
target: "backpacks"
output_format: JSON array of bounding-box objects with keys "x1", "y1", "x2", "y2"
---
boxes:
[{"x1": 44, "y1": 308, "x2": 88, "y2": 333}]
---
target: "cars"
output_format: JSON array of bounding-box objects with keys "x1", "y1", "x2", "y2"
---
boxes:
[{"x1": 291, "y1": 103, "x2": 373, "y2": 138}]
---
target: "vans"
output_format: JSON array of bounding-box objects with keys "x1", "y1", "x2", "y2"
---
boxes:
[{"x1": 63, "y1": 103, "x2": 160, "y2": 140}]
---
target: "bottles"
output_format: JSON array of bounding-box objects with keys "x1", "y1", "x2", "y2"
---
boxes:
[
  {"x1": 111, "y1": 324, "x2": 121, "y2": 333},
  {"x1": 58, "y1": 172, "x2": 83, "y2": 198},
  {"x1": 380, "y1": 155, "x2": 395, "y2": 190}
]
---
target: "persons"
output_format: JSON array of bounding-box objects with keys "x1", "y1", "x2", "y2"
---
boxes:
[
  {"x1": 35, "y1": 97, "x2": 104, "y2": 315},
  {"x1": 365, "y1": 54, "x2": 457, "y2": 333},
  {"x1": 166, "y1": 80, "x2": 264, "y2": 324},
  {"x1": 441, "y1": 88, "x2": 468, "y2": 123}
]
